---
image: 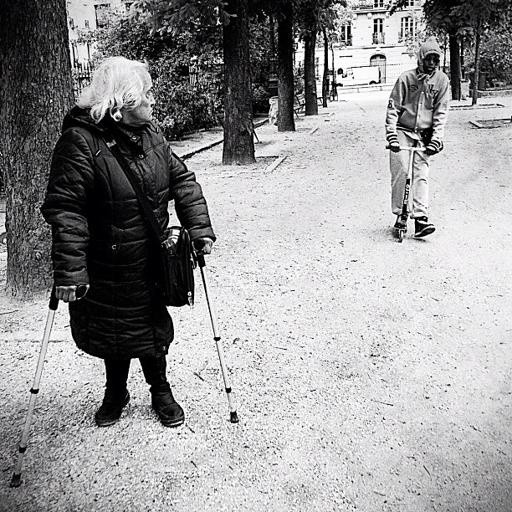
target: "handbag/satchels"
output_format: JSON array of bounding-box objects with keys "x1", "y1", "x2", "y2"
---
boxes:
[{"x1": 160, "y1": 225, "x2": 195, "y2": 308}]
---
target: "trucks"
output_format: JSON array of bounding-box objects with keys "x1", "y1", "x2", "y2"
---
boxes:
[{"x1": 335, "y1": 65, "x2": 381, "y2": 88}]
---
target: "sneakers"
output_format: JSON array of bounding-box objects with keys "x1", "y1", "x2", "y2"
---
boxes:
[
  {"x1": 148, "y1": 383, "x2": 186, "y2": 426},
  {"x1": 414, "y1": 216, "x2": 436, "y2": 237},
  {"x1": 94, "y1": 386, "x2": 130, "y2": 427},
  {"x1": 394, "y1": 215, "x2": 408, "y2": 231}
]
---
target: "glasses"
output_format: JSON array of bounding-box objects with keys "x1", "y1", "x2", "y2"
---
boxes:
[{"x1": 424, "y1": 56, "x2": 440, "y2": 64}]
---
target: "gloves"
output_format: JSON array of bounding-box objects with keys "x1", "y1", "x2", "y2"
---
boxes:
[
  {"x1": 426, "y1": 140, "x2": 440, "y2": 155},
  {"x1": 388, "y1": 135, "x2": 400, "y2": 151}
]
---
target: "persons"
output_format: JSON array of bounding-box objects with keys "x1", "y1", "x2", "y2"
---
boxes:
[
  {"x1": 41, "y1": 57, "x2": 219, "y2": 427},
  {"x1": 383, "y1": 37, "x2": 452, "y2": 240}
]
